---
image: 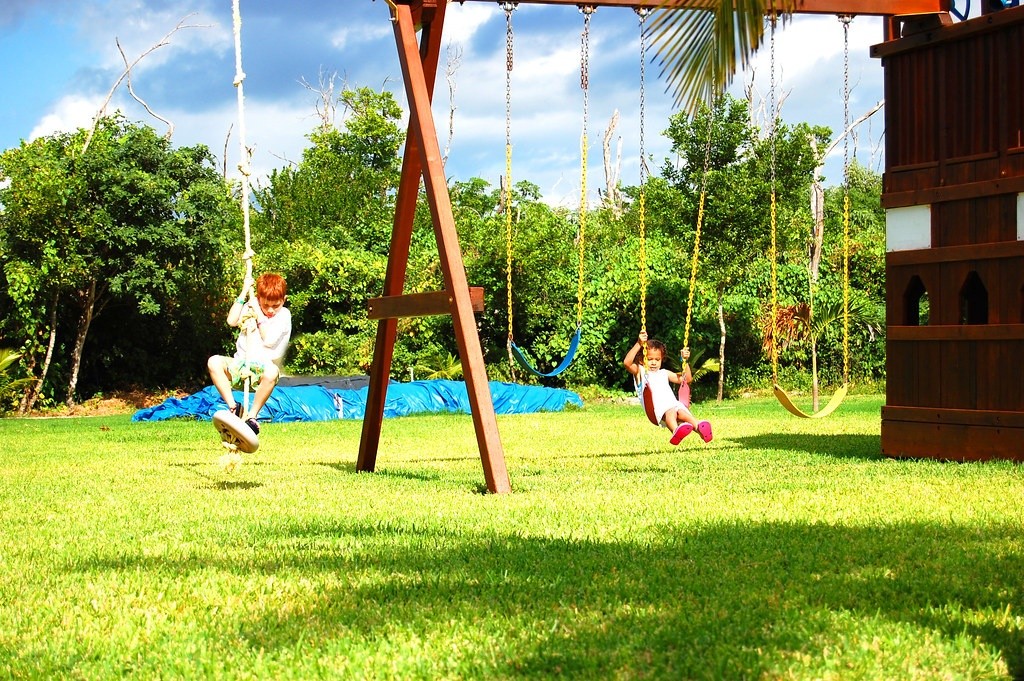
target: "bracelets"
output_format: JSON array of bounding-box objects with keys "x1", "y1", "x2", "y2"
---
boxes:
[
  {"x1": 235, "y1": 298, "x2": 246, "y2": 306},
  {"x1": 256, "y1": 316, "x2": 268, "y2": 328}
]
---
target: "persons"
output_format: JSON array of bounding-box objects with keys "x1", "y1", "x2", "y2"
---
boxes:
[
  {"x1": 624, "y1": 330, "x2": 713, "y2": 445},
  {"x1": 207, "y1": 273, "x2": 292, "y2": 435}
]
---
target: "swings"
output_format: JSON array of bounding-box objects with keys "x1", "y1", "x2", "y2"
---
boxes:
[
  {"x1": 495, "y1": 1, "x2": 598, "y2": 379},
  {"x1": 766, "y1": 11, "x2": 857, "y2": 420},
  {"x1": 631, "y1": 4, "x2": 722, "y2": 412}
]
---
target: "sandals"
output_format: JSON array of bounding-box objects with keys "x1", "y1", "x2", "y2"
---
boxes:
[
  {"x1": 245, "y1": 415, "x2": 259, "y2": 435},
  {"x1": 229, "y1": 400, "x2": 241, "y2": 417}
]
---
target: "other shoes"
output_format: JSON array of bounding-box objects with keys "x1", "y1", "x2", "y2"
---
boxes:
[
  {"x1": 697, "y1": 420, "x2": 713, "y2": 443},
  {"x1": 670, "y1": 422, "x2": 693, "y2": 445}
]
